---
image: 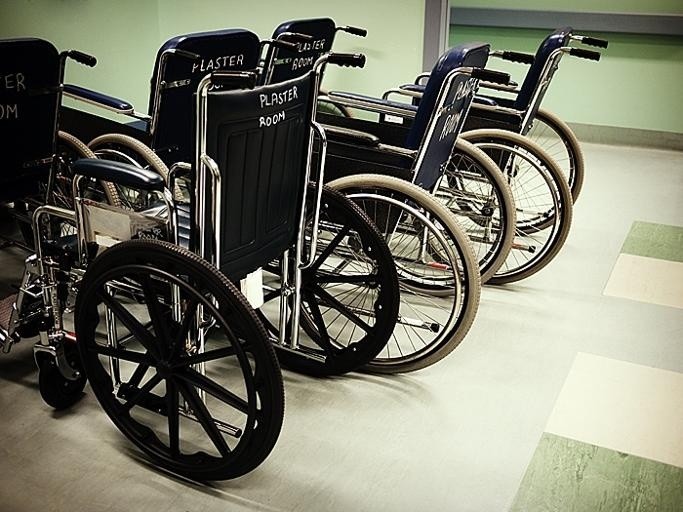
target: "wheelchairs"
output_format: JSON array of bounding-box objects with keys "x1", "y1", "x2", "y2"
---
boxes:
[
  {"x1": 376, "y1": 24, "x2": 608, "y2": 286},
  {"x1": 308, "y1": 38, "x2": 539, "y2": 377},
  {"x1": 0, "y1": 17, "x2": 401, "y2": 487}
]
